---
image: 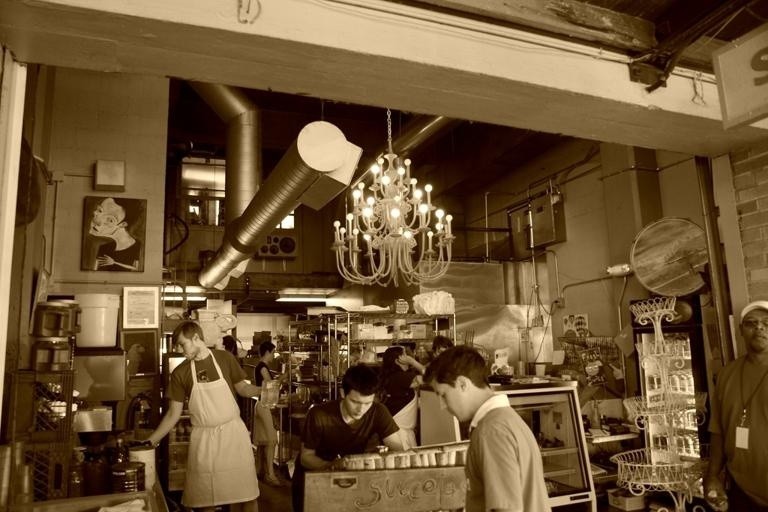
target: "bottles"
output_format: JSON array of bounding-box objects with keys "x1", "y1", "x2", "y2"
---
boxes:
[
  {"x1": 0, "y1": 441, "x2": 35, "y2": 512},
  {"x1": 709, "y1": 491, "x2": 729, "y2": 512}
]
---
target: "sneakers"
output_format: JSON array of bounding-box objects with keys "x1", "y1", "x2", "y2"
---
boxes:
[{"x1": 256, "y1": 471, "x2": 279, "y2": 487}]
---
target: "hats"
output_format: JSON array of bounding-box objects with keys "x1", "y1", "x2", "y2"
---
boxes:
[{"x1": 740, "y1": 300, "x2": 767, "y2": 318}]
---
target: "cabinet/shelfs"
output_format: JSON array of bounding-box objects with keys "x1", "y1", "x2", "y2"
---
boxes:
[{"x1": 418, "y1": 380, "x2": 597, "y2": 511}]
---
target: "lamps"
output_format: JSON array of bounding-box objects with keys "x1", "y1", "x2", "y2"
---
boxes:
[{"x1": 330, "y1": 109, "x2": 457, "y2": 289}]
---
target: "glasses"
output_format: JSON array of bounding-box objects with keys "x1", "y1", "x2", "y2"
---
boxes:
[{"x1": 743, "y1": 320, "x2": 768, "y2": 327}]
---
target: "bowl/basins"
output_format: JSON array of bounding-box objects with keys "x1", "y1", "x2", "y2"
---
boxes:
[{"x1": 314, "y1": 333, "x2": 328, "y2": 343}]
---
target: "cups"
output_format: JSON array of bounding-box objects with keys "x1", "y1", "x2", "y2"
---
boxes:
[{"x1": 261, "y1": 380, "x2": 280, "y2": 410}]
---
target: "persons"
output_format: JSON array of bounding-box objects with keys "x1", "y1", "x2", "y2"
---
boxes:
[
  {"x1": 701, "y1": 299, "x2": 768, "y2": 511},
  {"x1": 425, "y1": 343, "x2": 551, "y2": 512},
  {"x1": 139, "y1": 321, "x2": 261, "y2": 512},
  {"x1": 290, "y1": 364, "x2": 406, "y2": 512},
  {"x1": 253, "y1": 341, "x2": 289, "y2": 487},
  {"x1": 424, "y1": 336, "x2": 452, "y2": 368},
  {"x1": 371, "y1": 346, "x2": 426, "y2": 449},
  {"x1": 84, "y1": 197, "x2": 142, "y2": 272}
]
